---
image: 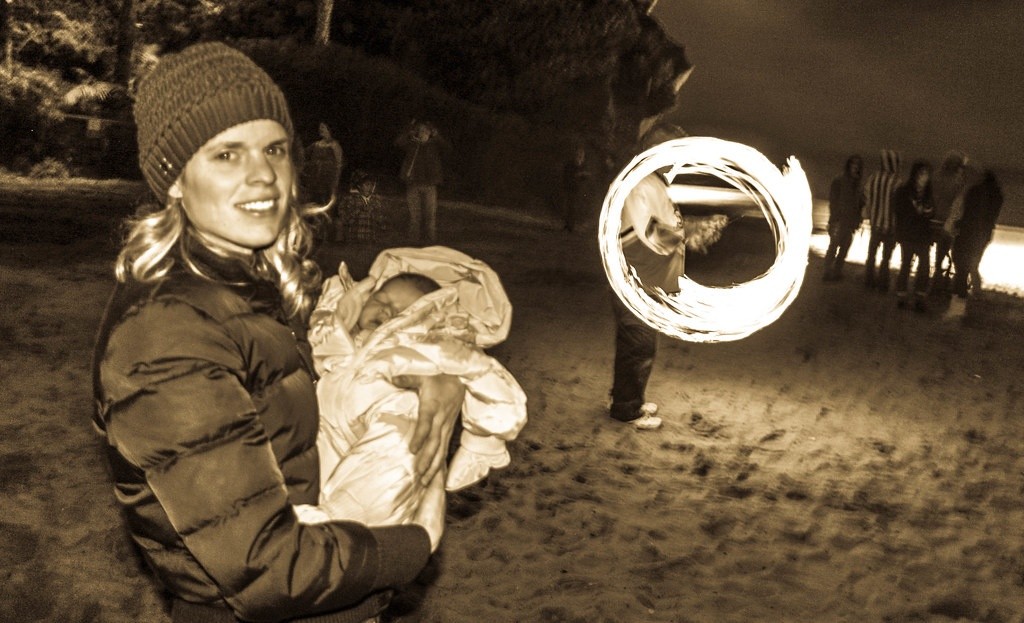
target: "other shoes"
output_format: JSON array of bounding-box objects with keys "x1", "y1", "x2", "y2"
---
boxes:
[
  {"x1": 606, "y1": 395, "x2": 660, "y2": 417},
  {"x1": 626, "y1": 413, "x2": 663, "y2": 430}
]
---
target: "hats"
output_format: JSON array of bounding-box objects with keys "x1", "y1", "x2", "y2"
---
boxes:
[{"x1": 132, "y1": 41, "x2": 295, "y2": 207}]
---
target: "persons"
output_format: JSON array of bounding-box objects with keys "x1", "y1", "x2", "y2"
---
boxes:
[
  {"x1": 302, "y1": 115, "x2": 446, "y2": 247},
  {"x1": 823, "y1": 149, "x2": 1004, "y2": 318},
  {"x1": 91, "y1": 41, "x2": 475, "y2": 623},
  {"x1": 608, "y1": 122, "x2": 729, "y2": 430},
  {"x1": 316, "y1": 246, "x2": 527, "y2": 525}
]
